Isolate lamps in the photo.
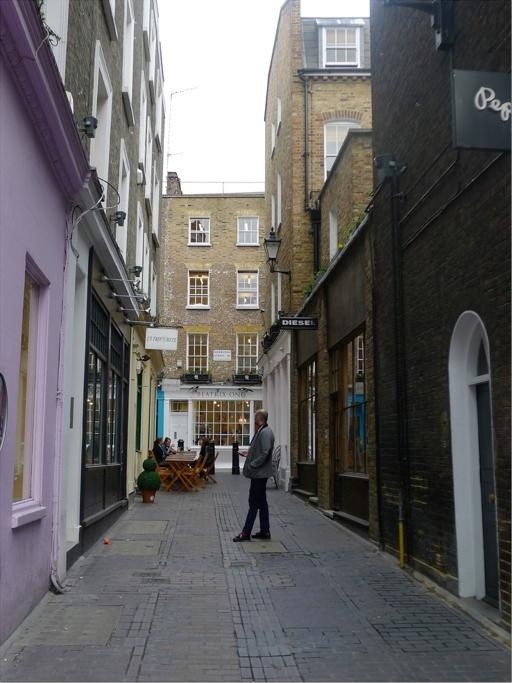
[263,226,290,280]
[76,114,99,143]
[109,210,127,226]
[138,354,152,362]
[101,265,157,328]
[373,153,403,172]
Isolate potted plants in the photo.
[231,370,260,382]
[264,320,279,346]
[182,368,212,381]
[137,458,161,503]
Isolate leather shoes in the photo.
[252,531,270,539]
[233,532,250,542]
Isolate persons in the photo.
[152,437,170,467]
[189,436,213,479]
[159,437,177,461]
[231,408,275,542]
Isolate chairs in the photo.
[148,449,219,493]
